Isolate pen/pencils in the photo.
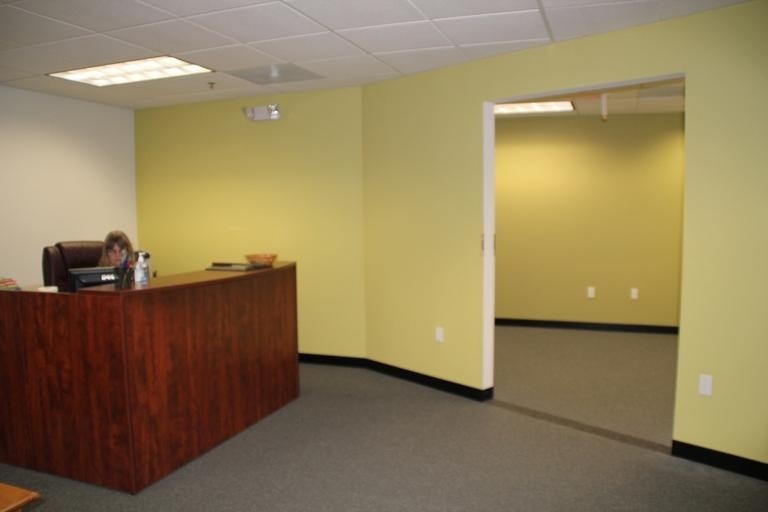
[212,262,233,266]
[120,248,134,289]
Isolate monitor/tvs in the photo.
[67,266,135,293]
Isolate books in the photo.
[206,264,273,272]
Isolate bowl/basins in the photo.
[246,253,278,269]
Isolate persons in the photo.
[98,230,134,267]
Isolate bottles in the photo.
[133,252,149,285]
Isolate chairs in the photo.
[42,241,104,291]
